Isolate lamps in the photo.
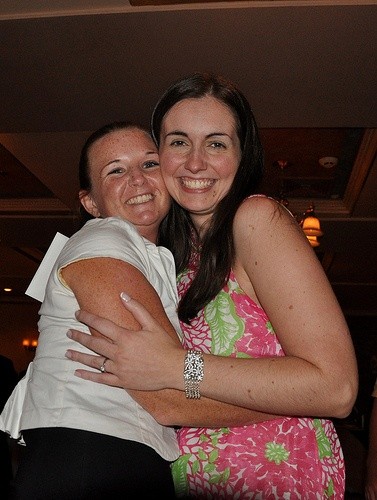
[301,200,324,247]
